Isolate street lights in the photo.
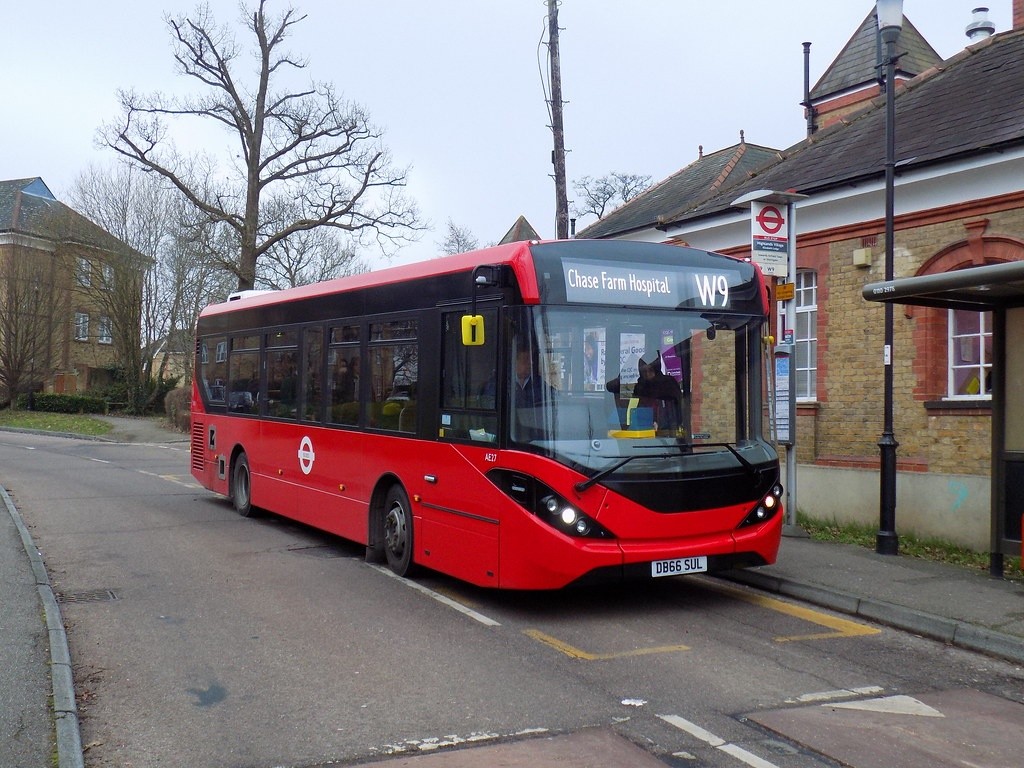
[876,0,909,555]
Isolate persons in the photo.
[586,336,599,376]
[249,342,685,447]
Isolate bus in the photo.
[189,237,785,591]
